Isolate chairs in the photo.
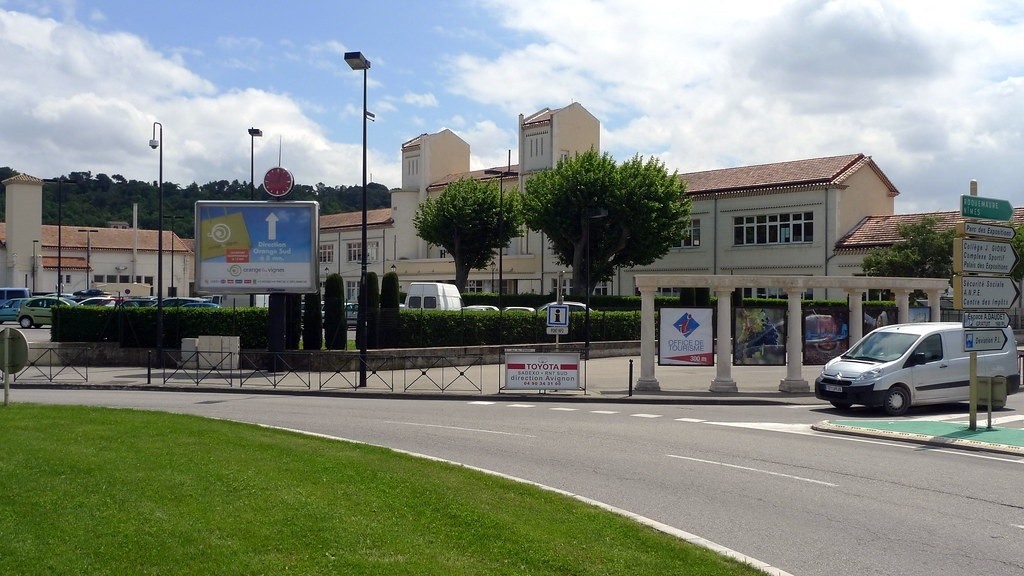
[926,339,940,359]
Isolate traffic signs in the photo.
[952,194,1021,352]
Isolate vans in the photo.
[815,321,1020,416]
[404,281,465,310]
[0,288,32,308]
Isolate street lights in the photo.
[78,230,98,290]
[42,179,78,298]
[163,215,184,298]
[345,51,371,387]
[32,240,39,292]
[584,208,609,359]
[248,127,263,306]
[484,169,519,344]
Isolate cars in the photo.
[344,303,360,329]
[0,298,29,324]
[16,296,79,328]
[151,295,223,307]
[300,301,326,329]
[538,301,594,312]
[67,295,165,307]
[504,307,535,312]
[462,305,499,311]
